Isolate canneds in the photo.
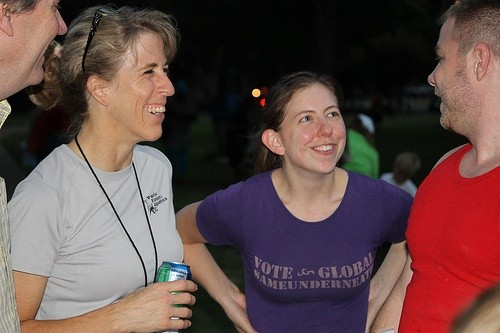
[152,260,192,321]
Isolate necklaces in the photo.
[74,132,158,288]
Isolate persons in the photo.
[176,71,419,333]
[369,0,500,333]
[0,0,69,333]
[341,109,419,199]
[4,4,198,332]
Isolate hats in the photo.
[359,114,374,134]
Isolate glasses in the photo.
[82,6,121,80]
